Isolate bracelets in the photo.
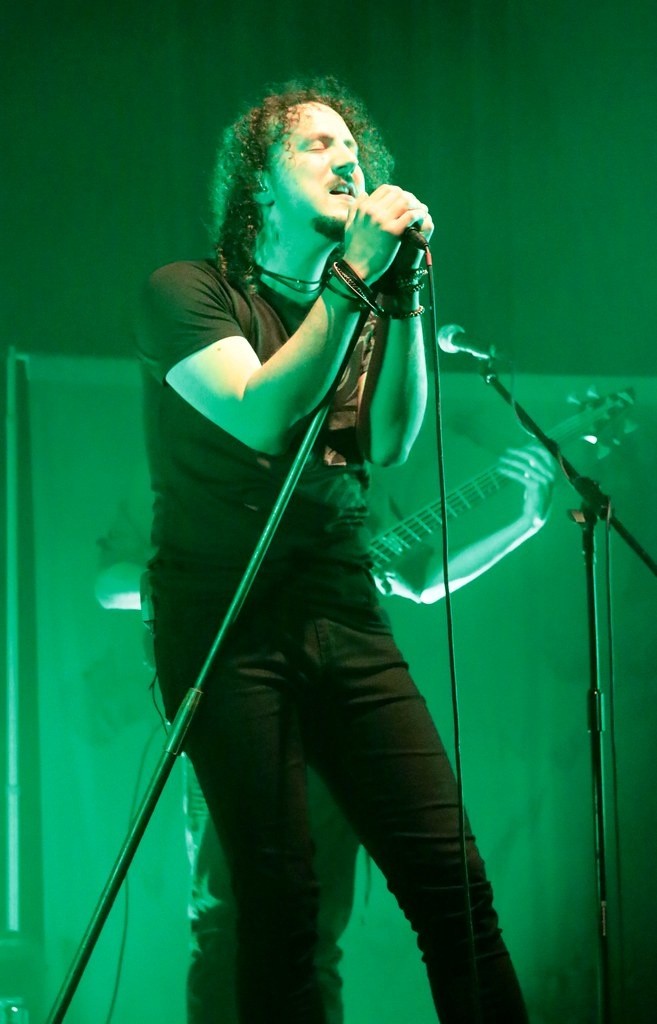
[375,268,427,320]
[326,259,375,307]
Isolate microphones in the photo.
[438,324,494,362]
[353,192,428,250]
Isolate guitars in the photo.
[140,383,642,675]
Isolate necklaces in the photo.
[259,267,324,294]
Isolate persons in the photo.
[95,443,559,1024]
[132,93,532,1024]
